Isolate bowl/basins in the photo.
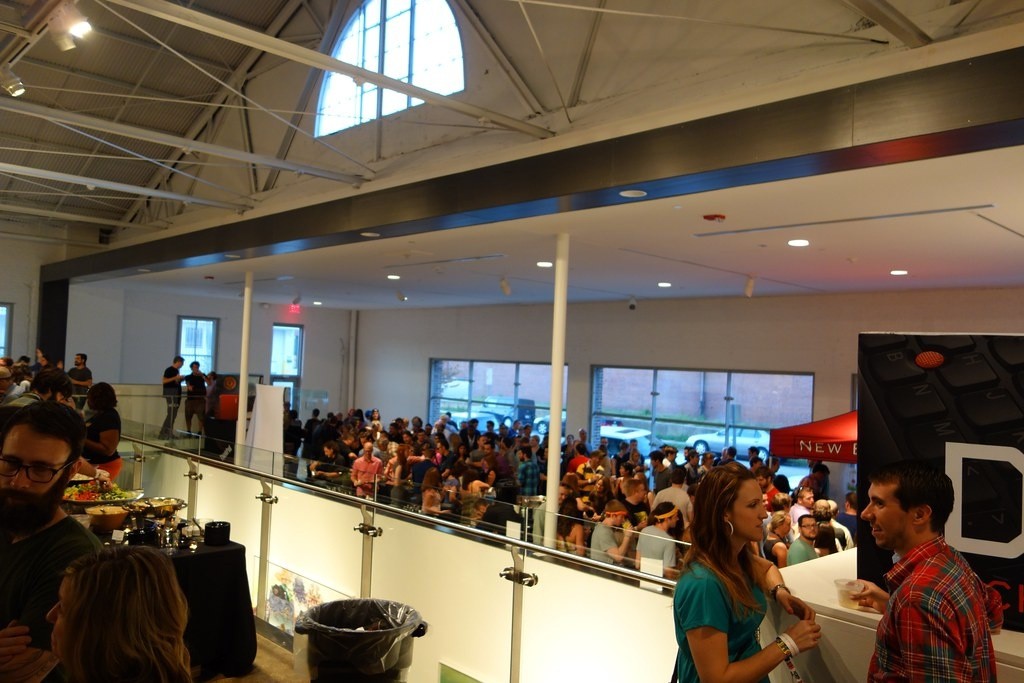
[204,521,230,545]
[60,491,144,515]
[85,505,129,529]
[122,496,187,519]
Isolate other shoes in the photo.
[184,433,191,437]
[195,432,201,438]
[158,434,178,439]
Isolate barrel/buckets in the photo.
[219,395,237,419]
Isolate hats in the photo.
[686,450,703,457]
[365,410,373,416]
[0,366,11,378]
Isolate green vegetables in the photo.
[63,482,131,499]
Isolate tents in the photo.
[769,410,858,469]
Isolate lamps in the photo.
[743,276,756,298]
[45,2,93,51]
[499,278,512,296]
[395,290,407,301]
[0,61,25,97]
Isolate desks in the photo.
[88,516,257,676]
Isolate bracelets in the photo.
[95,470,100,479]
[776,633,802,683]
[772,585,791,602]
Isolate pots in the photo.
[66,479,91,487]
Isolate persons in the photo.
[0,349,123,481]
[748,446,858,567]
[185,361,216,439]
[159,356,185,440]
[533,429,737,592]
[673,461,821,683]
[47,546,193,683]
[843,460,998,683]
[0,401,102,683]
[284,401,549,548]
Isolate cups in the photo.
[622,518,633,530]
[639,512,647,521]
[835,579,865,609]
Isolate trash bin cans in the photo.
[295,597,428,683]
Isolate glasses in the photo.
[0,458,76,483]
[800,523,818,529]
